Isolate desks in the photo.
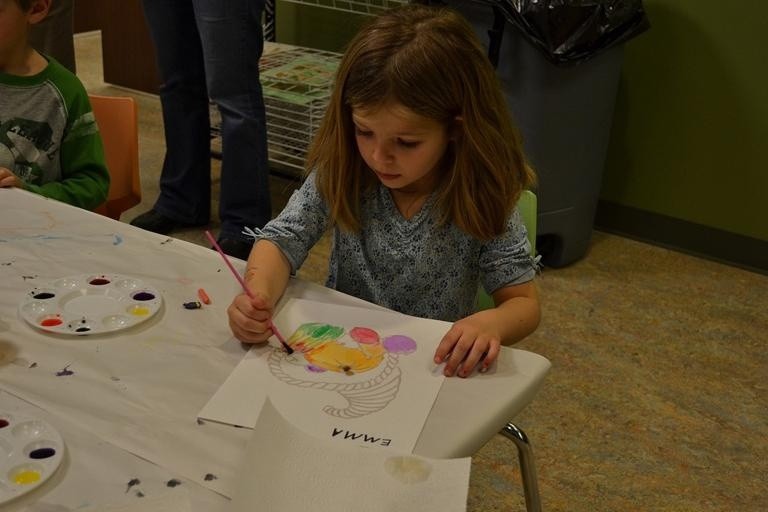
[0,186,551,512]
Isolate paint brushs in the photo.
[205,231,294,354]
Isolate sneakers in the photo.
[210,229,254,264]
[129,209,213,234]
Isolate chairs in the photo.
[87,94,142,221]
[466,191,542,512]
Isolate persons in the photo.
[128,0,274,262]
[227,9,541,377]
[0,0,113,211]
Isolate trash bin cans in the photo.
[447,0,652,269]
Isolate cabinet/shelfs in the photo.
[210,42,344,171]
